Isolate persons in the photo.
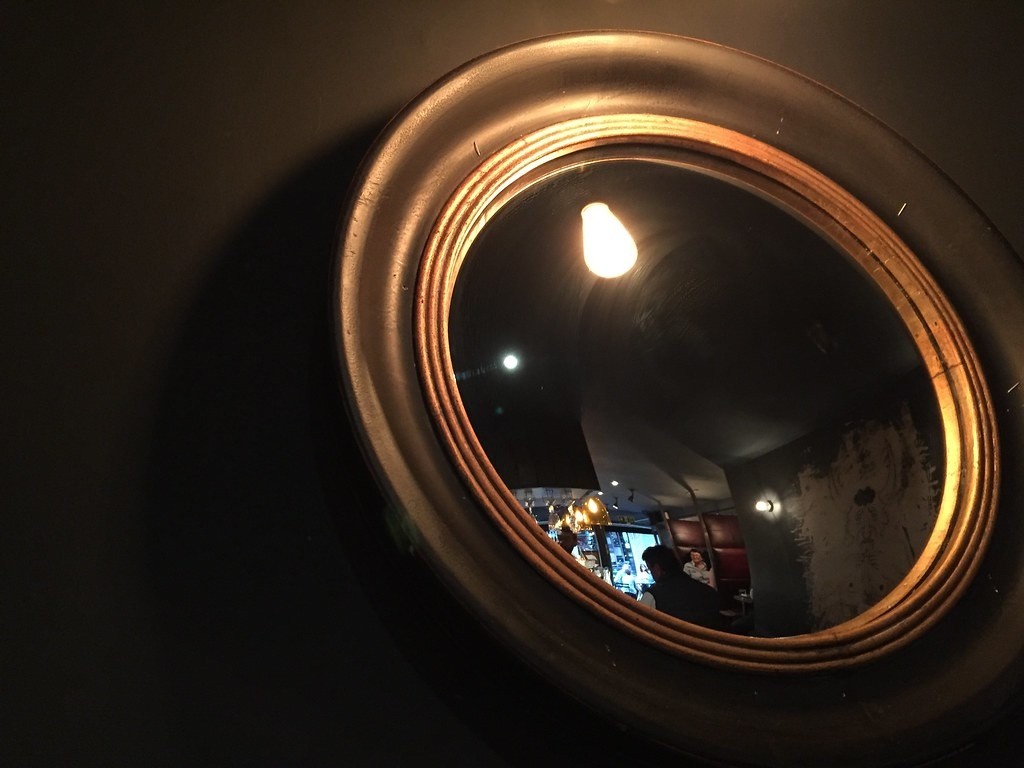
[639,544,721,631]
[613,562,655,601]
[701,549,717,590]
[683,548,708,584]
